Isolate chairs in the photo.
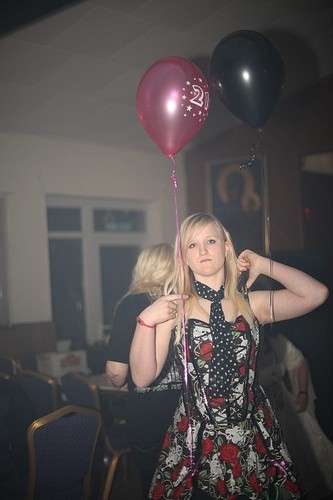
[0,358,144,500]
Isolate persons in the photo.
[105,243,182,500]
[128,212,329,500]
[256,332,333,500]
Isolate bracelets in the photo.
[299,391,307,394]
[136,316,156,328]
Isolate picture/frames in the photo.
[204,154,271,259]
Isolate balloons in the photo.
[209,29,284,129]
[136,57,211,158]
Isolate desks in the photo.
[28,349,95,402]
[85,368,182,396]
